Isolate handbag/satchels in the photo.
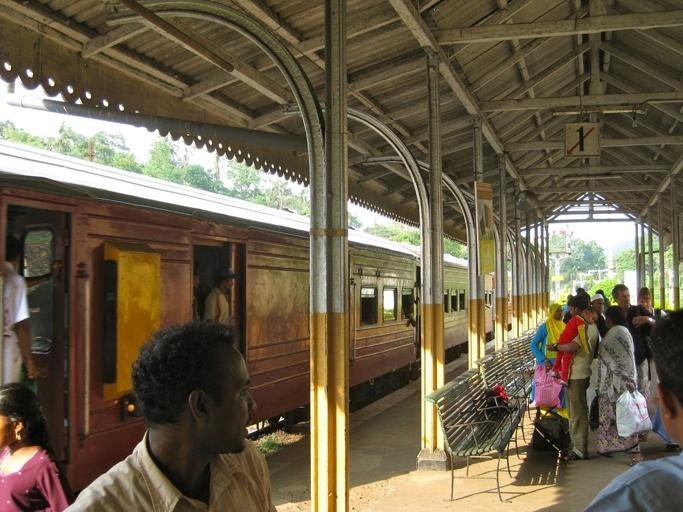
[533,416,570,449]
[534,365,563,409]
[589,397,599,431]
[616,389,652,437]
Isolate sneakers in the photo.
[563,452,581,461]
[629,454,642,465]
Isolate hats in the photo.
[217,267,239,279]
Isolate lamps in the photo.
[632,105,638,128]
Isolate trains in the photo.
[0,137,494,500]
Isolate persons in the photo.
[0,381,69,511]
[204,267,234,327]
[530,284,683,462]
[582,308,683,512]
[193,266,199,320]
[0,234,61,390]
[62,319,277,511]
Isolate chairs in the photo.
[423,326,536,502]
